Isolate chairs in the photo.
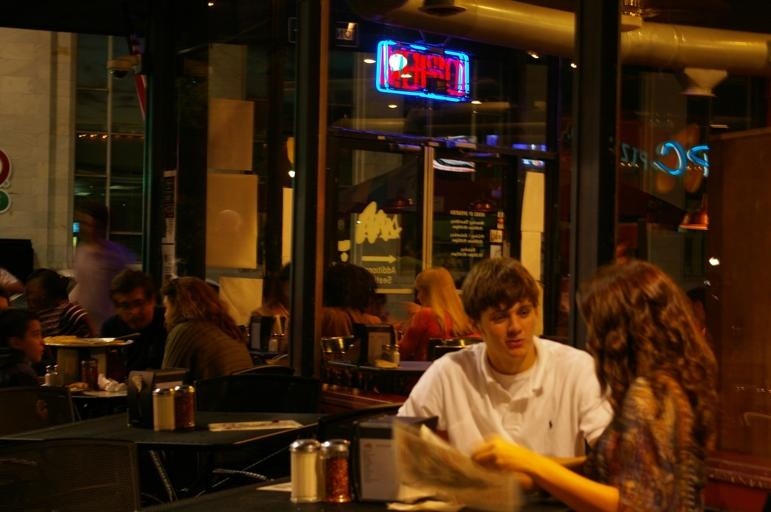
[317,401,438,443]
[351,323,399,364]
[230,363,297,376]
[190,374,324,413]
[0,384,76,437]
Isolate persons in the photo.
[468,259,721,512]
[616,244,634,259]
[2,208,398,512]
[399,266,472,359]
[394,257,606,459]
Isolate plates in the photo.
[43,337,134,347]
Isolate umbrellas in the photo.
[334,141,690,225]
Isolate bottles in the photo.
[152,387,176,432]
[268,334,278,352]
[320,439,351,504]
[53,364,64,387]
[278,334,288,353]
[391,344,400,366]
[174,385,195,428]
[44,365,55,385]
[289,439,322,504]
[81,358,98,388]
[380,345,391,362]
